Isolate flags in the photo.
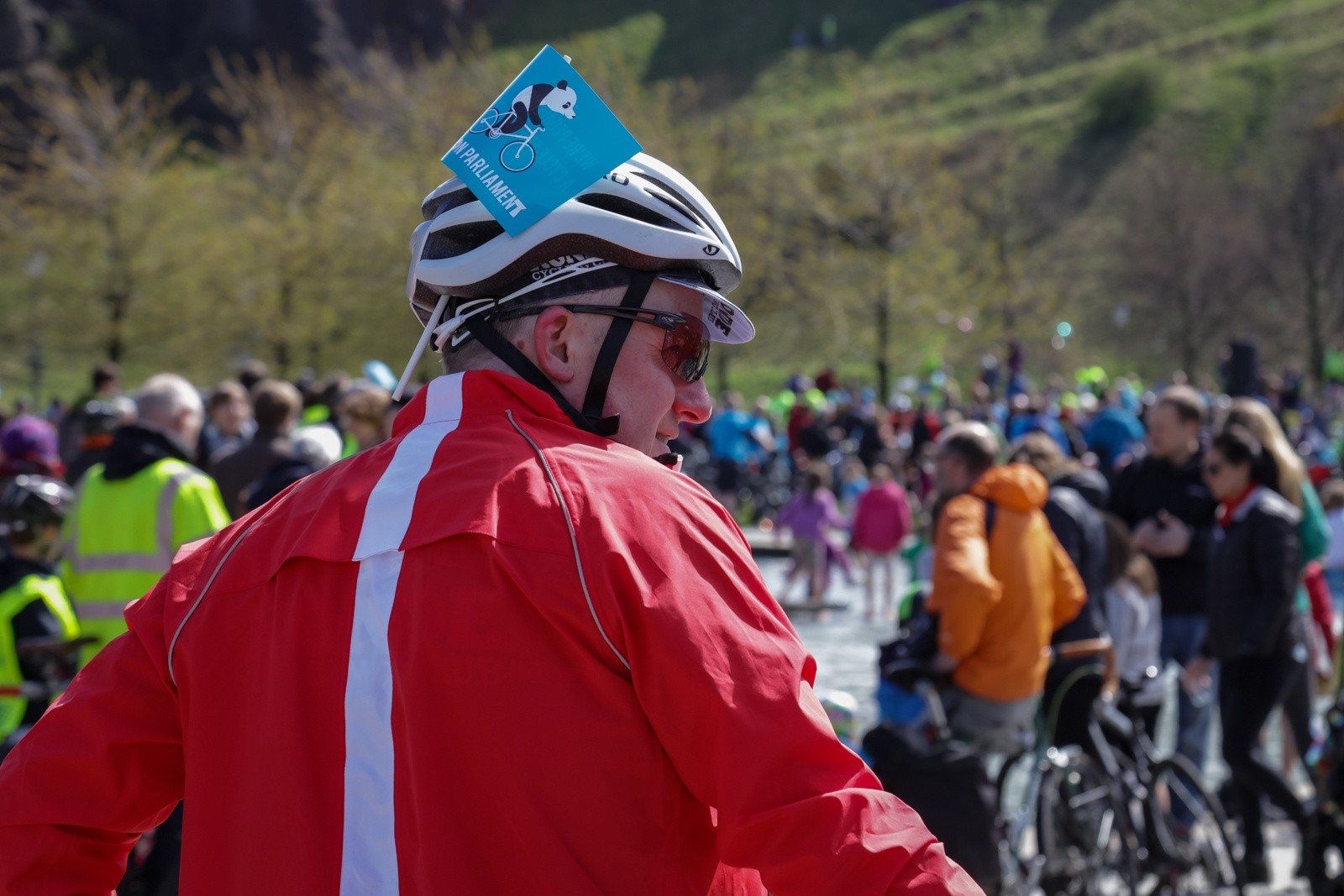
[440,44,644,237]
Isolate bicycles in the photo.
[857,625,1344,896]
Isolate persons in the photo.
[1,152,984,896]
[667,335,1344,896]
[1,360,411,896]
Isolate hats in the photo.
[436,250,755,345]
[0,414,61,472]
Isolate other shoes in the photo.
[1213,855,1269,886]
[1293,848,1312,879]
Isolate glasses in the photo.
[498,305,712,384]
[1201,458,1230,478]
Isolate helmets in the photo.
[0,473,74,545]
[407,151,742,351]
